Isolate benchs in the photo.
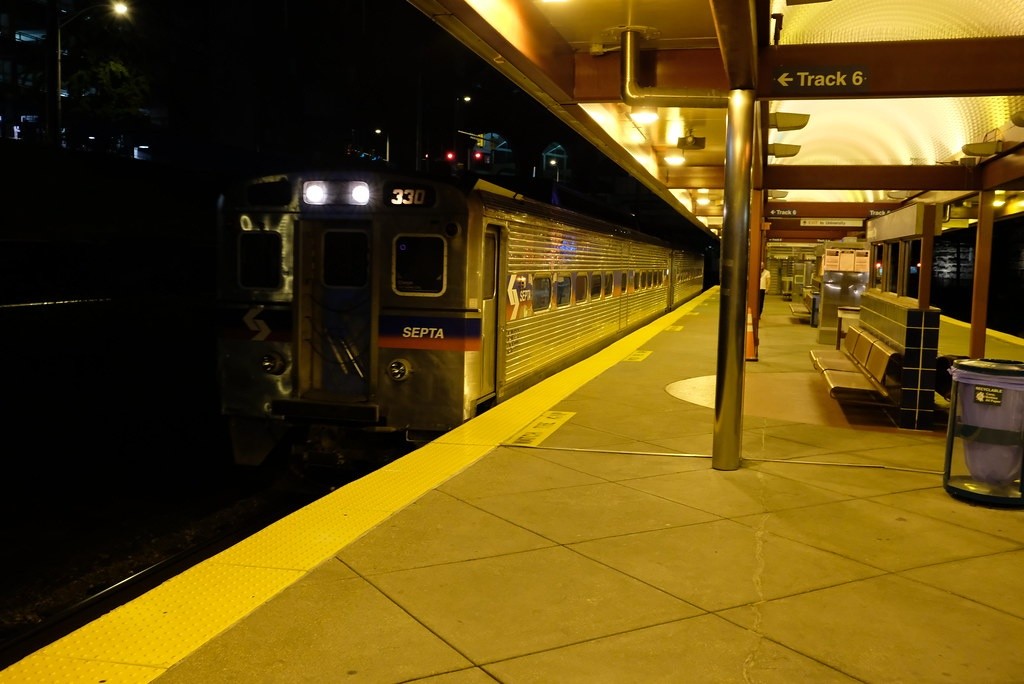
[790,293,816,325]
[810,326,902,410]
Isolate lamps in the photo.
[677,136,706,151]
[768,143,801,158]
[886,192,905,200]
[769,112,810,132]
[961,140,1003,157]
[767,190,788,198]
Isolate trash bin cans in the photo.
[941,355,1024,511]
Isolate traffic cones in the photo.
[744,306,758,362]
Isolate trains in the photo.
[215,168,703,450]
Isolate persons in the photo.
[759,262,771,319]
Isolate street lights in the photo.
[55,1,130,120]
[375,127,390,162]
